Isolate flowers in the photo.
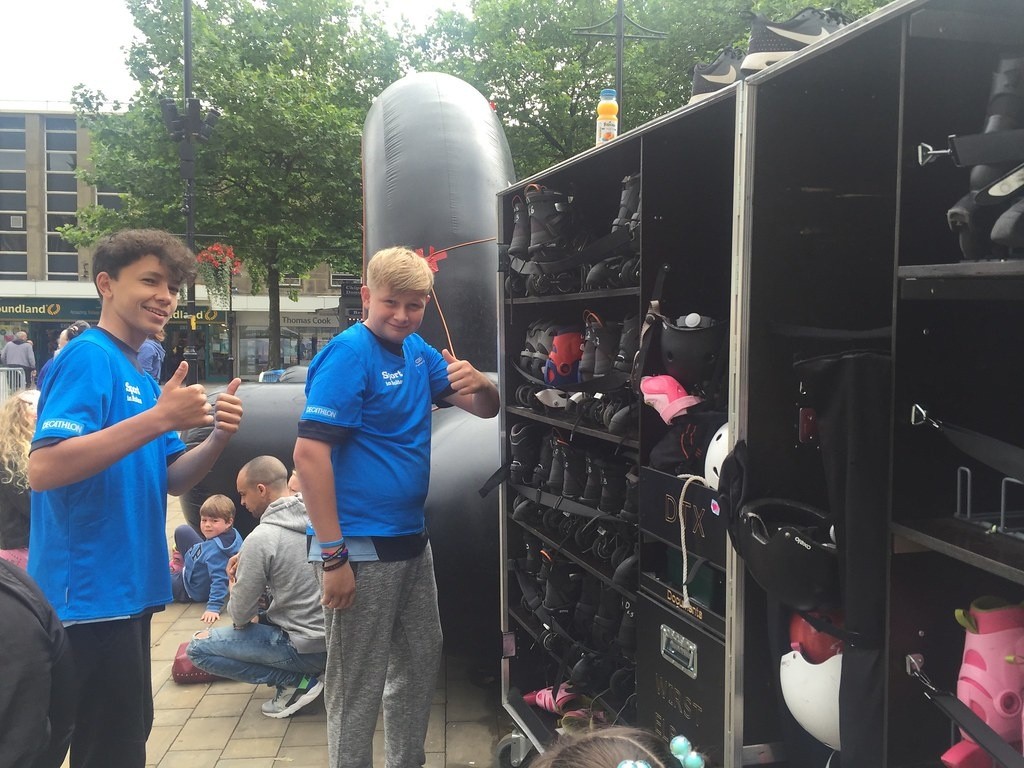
[198,242,242,311]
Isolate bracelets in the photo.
[318,537,348,571]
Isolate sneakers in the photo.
[507,5,1024,768]
[261,674,324,719]
[173,544,185,565]
[169,560,183,574]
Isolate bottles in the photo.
[596,88,619,148]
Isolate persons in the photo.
[25,228,243,768]
[287,470,301,496]
[292,245,501,768]
[137,321,165,384]
[0,558,77,768]
[0,390,43,571]
[166,495,243,623]
[56,321,90,350]
[1,331,37,393]
[186,456,327,719]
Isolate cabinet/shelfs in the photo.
[492,0,1024,768]
